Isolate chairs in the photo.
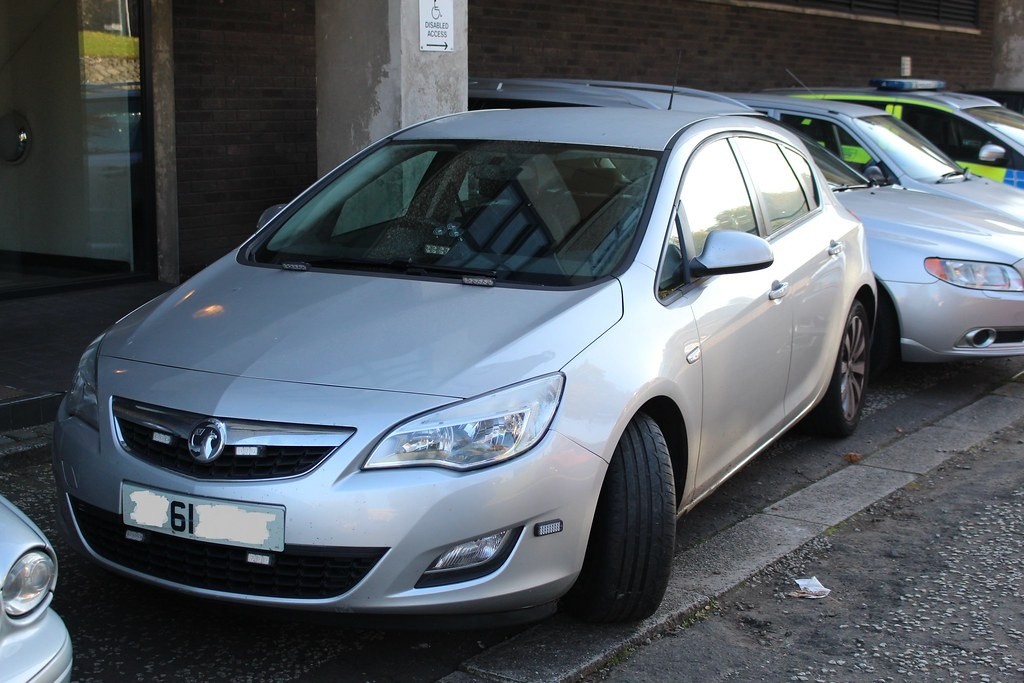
[458,155,566,257]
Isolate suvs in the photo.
[468,74,1023,383]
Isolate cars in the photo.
[46,103,880,633]
[710,91,1022,224]
[763,89,1023,190]
[0,495,75,683]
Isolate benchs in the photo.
[537,163,643,250]
